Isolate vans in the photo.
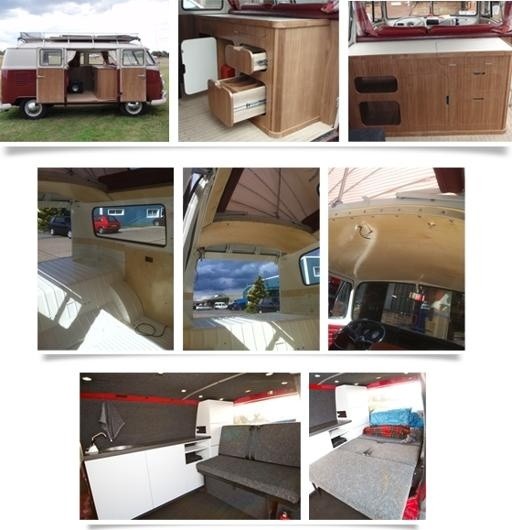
[214,302,229,310]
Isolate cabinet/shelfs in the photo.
[179,12,337,139]
[92,65,118,99]
[83,435,212,519]
[347,36,512,138]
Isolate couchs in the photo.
[198,421,300,519]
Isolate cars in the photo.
[47,215,72,239]
[255,302,280,313]
[93,215,121,234]
[195,304,213,310]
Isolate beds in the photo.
[309,433,423,520]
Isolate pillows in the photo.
[364,408,424,442]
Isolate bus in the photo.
[1,32,166,119]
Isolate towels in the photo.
[100,400,124,440]
